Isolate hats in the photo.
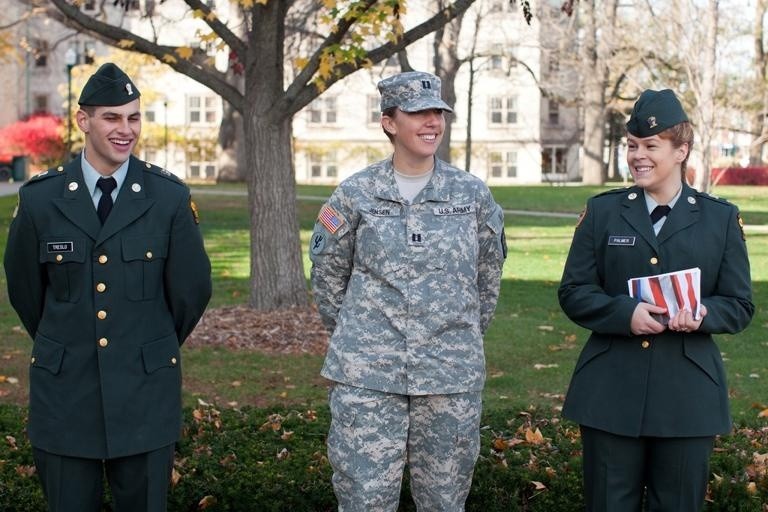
[378,71,454,113]
[78,63,140,107]
[627,89,689,138]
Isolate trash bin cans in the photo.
[12,156,25,181]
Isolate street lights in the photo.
[62,46,80,165]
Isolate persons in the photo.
[309,70,509,511]
[3,62,213,512]
[556,87,756,512]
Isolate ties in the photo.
[96,177,117,224]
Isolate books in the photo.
[627,267,702,328]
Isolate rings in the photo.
[680,327,688,332]
